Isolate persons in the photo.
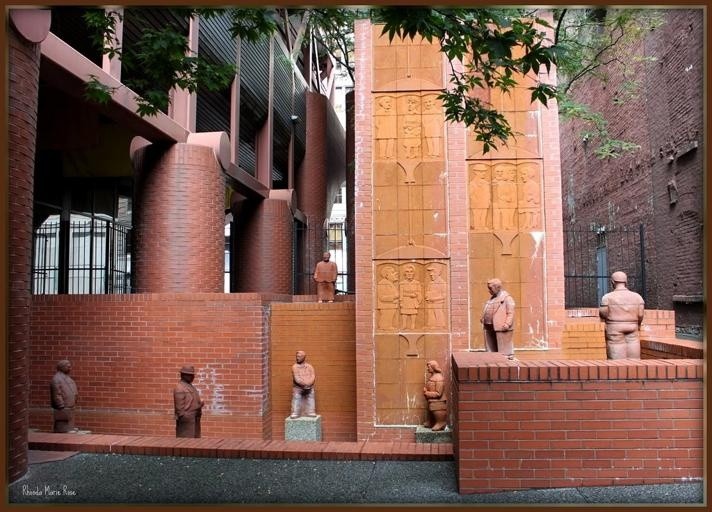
[175,363,204,437]
[315,252,338,304]
[479,277,515,353]
[599,271,644,360]
[50,358,80,432]
[423,360,449,431]
[290,348,317,417]
